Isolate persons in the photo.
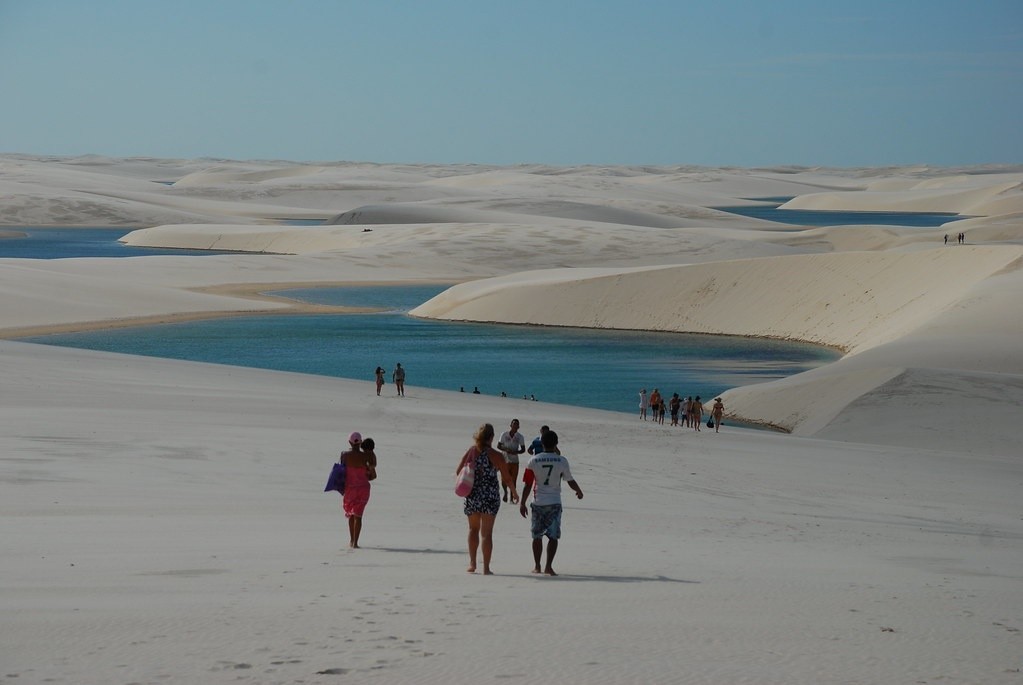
[374,366,385,395]
[527,425,560,455]
[649,389,660,422]
[497,419,525,503]
[944,235,947,244]
[659,393,704,431]
[473,387,480,394]
[340,432,377,548]
[456,424,519,576]
[501,392,506,397]
[711,397,724,433]
[460,387,465,392]
[958,233,964,244]
[530,395,534,401]
[393,363,405,395]
[639,389,648,421]
[523,394,526,399]
[520,431,583,576]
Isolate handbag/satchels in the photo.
[323,451,346,496]
[706,419,714,428]
[454,446,475,497]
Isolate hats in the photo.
[695,396,702,400]
[714,398,722,402]
[349,432,363,444]
